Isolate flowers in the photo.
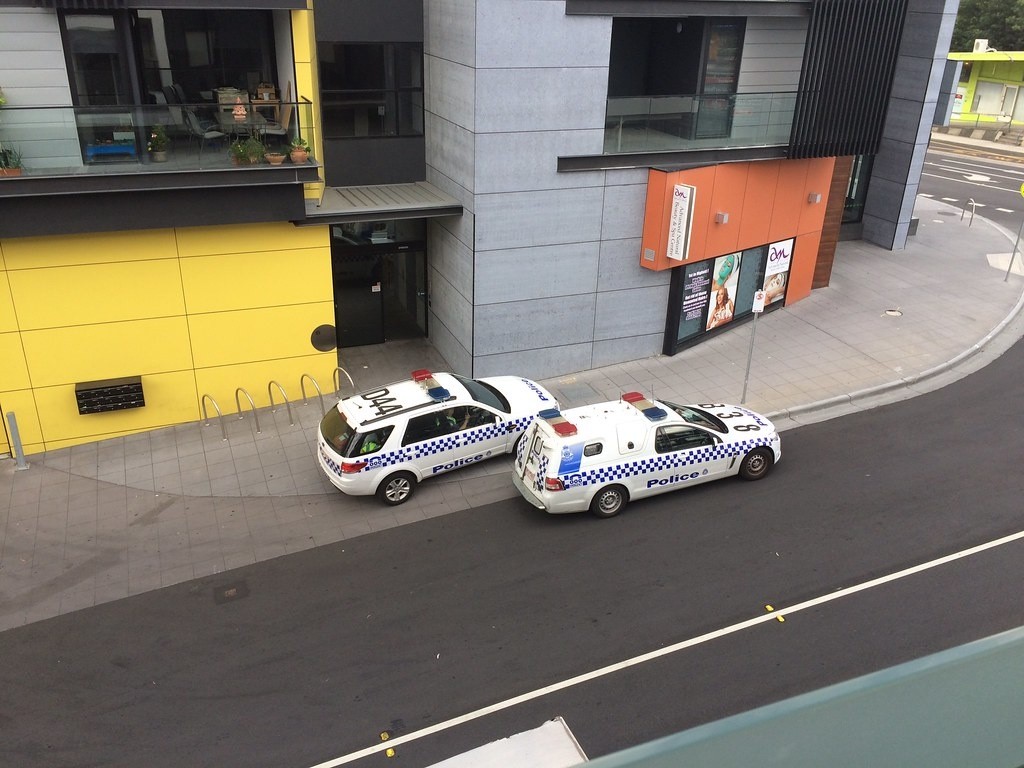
[227,136,266,165]
[147,124,173,152]
[233,154,241,165]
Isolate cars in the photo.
[316,367,560,507]
[515,392,782,519]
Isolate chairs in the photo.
[256,82,293,146]
[162,82,231,161]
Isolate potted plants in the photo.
[286,137,311,164]
[0,139,30,177]
[264,144,286,165]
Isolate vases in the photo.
[153,149,167,163]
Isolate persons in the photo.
[763,273,786,306]
[706,253,739,330]
[434,408,470,431]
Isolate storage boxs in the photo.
[257,83,276,99]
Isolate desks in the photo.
[212,88,250,113]
[215,111,267,147]
[252,98,282,122]
